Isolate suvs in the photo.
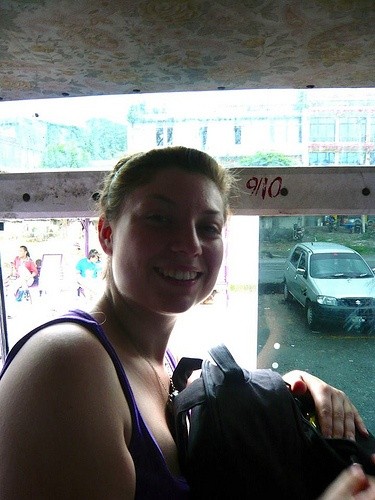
[282,242,375,334]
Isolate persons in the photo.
[6,246,38,302]
[72,248,101,311]
[0,146,375,500]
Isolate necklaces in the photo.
[105,295,180,398]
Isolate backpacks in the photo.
[163,342,375,500]
